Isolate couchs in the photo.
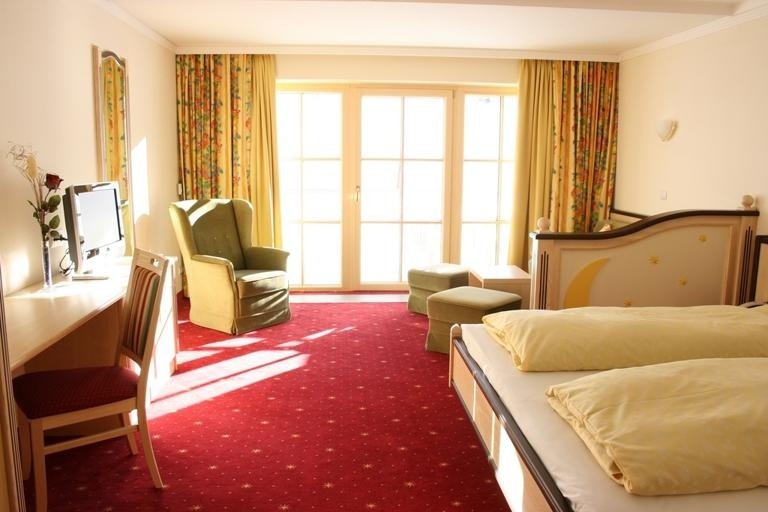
[528,195,758,310]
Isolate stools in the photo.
[424,285,522,355]
[407,262,467,315]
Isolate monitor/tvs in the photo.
[63,181,124,280]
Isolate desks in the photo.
[3,253,181,437]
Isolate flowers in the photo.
[6,140,64,281]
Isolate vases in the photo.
[41,240,53,288]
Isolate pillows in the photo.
[598,223,612,233]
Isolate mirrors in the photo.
[92,44,131,208]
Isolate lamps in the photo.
[656,117,677,143]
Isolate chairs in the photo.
[12,246,169,512]
[169,198,292,336]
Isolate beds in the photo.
[447,234,768,512]
